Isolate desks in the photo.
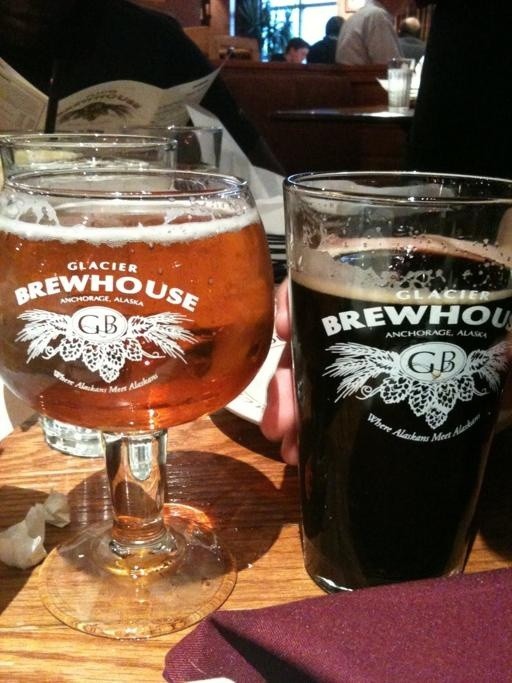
[270,104,415,143]
[1,413,511,683]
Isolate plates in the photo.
[224,317,288,425]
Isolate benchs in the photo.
[218,64,415,173]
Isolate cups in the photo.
[384,56,415,113]
[284,167,512,598]
[3,126,221,462]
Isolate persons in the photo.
[258,0,511,470]
[0,0,292,180]
[305,14,344,61]
[269,37,310,66]
[334,0,403,63]
[394,17,427,60]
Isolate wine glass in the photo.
[3,174,270,642]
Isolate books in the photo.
[0,51,233,140]
